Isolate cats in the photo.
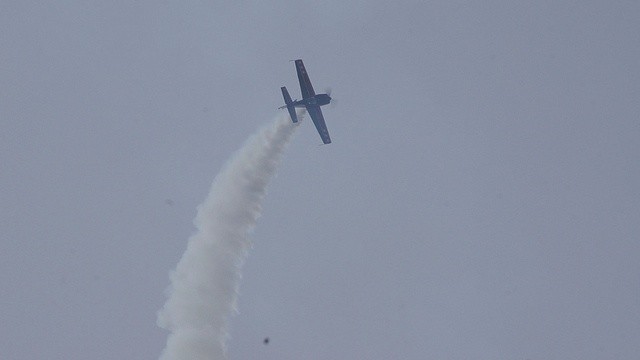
[278,58,335,145]
